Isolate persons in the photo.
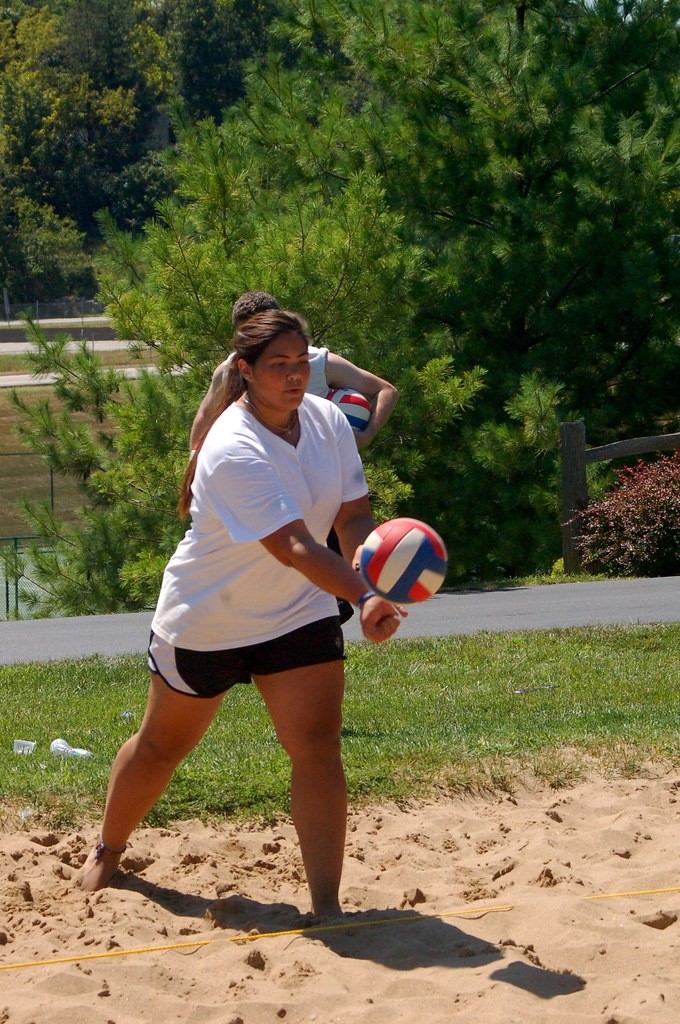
[189,290,398,624]
[80,308,408,924]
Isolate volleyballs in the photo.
[361,519,447,603]
[325,388,371,433]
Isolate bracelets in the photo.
[358,590,377,609]
